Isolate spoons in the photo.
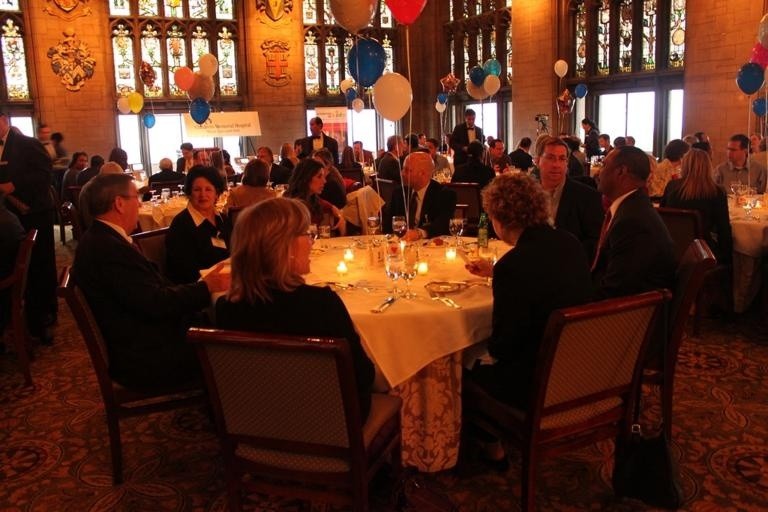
[373,296,394,314]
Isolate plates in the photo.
[425,282,459,293]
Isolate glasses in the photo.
[294,228,317,243]
[112,192,142,204]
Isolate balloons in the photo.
[370,73,414,122]
[117,51,218,127]
[554,59,588,117]
[737,15,768,115]
[383,0,427,25]
[331,0,379,32]
[338,76,367,111]
[350,39,386,87]
[436,57,503,113]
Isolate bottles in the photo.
[366,218,383,246]
[478,212,487,248]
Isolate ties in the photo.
[590,210,611,272]
[409,191,418,229]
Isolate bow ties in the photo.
[310,136,321,139]
[466,127,473,130]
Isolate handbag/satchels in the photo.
[612,434,681,512]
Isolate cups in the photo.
[590,155,605,166]
[146,184,186,209]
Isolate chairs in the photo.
[653,207,702,254]
[626,238,716,447]
[454,204,470,236]
[57,265,209,484]
[188,327,403,512]
[360,166,480,220]
[0,227,37,385]
[48,180,288,247]
[129,225,169,286]
[463,288,672,512]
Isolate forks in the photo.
[430,291,462,309]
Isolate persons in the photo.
[0,112,767,500]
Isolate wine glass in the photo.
[449,220,463,249]
[319,225,330,253]
[403,246,419,299]
[727,184,761,224]
[392,217,407,245]
[306,225,318,257]
[386,254,404,302]
